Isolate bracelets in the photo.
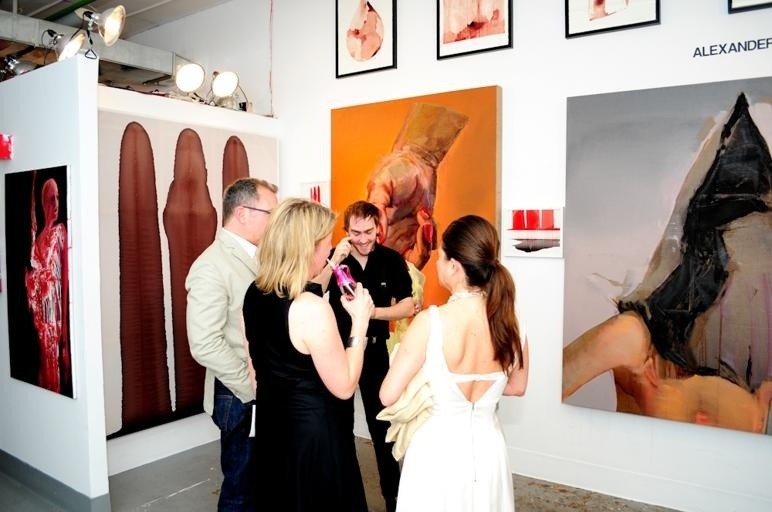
[344,334,368,351]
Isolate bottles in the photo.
[328,262,360,303]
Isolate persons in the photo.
[379,214,529,512]
[241,197,375,512]
[562,91,772,435]
[24,177,68,399]
[366,105,471,271]
[308,202,414,512]
[185,178,277,475]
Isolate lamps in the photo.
[37,29,75,66]
[208,71,250,109]
[0,54,39,75]
[79,5,126,58]
[171,64,206,101]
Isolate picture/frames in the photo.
[437,0,512,59]
[564,0,660,38]
[335,1,397,78]
[727,0,772,13]
